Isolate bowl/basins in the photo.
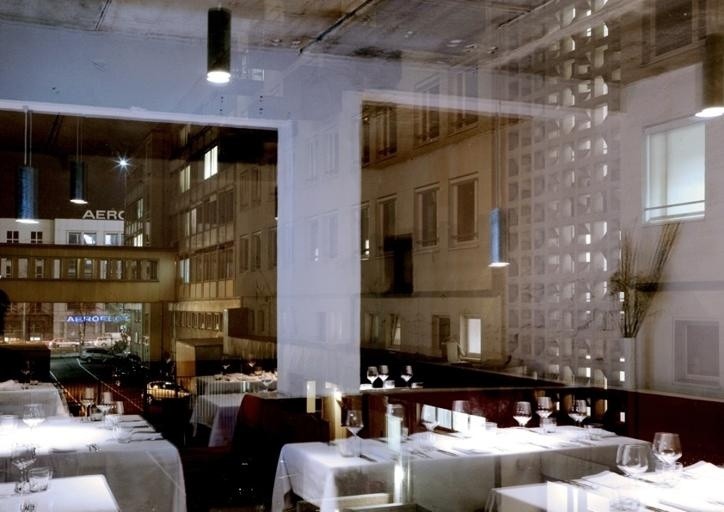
[146,380,176,391]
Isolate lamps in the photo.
[16,105,40,226]
[488,49,513,272]
[692,5,723,119]
[204,1,233,86]
[69,116,91,208]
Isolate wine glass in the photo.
[613,428,685,511]
[214,357,274,397]
[13,369,43,390]
[346,364,595,457]
[0,389,123,512]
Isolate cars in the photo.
[49,331,192,404]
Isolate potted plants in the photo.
[606,220,683,391]
